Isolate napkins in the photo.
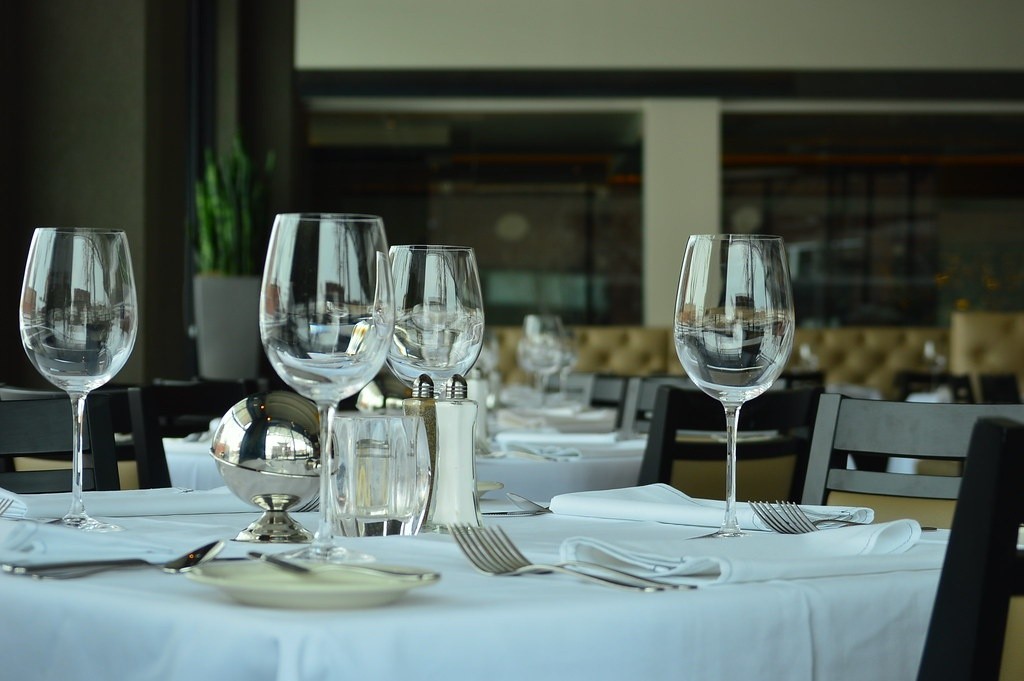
[534,444,583,464]
[0,522,178,568]
[558,537,731,592]
[549,482,875,532]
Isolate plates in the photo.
[188,564,441,611]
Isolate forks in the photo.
[477,526,699,589]
[747,500,800,534]
[1,498,14,516]
[446,523,665,591]
[775,499,856,533]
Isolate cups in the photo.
[329,412,420,537]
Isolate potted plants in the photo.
[193,124,278,378]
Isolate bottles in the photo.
[426,373,486,533]
[402,374,438,526]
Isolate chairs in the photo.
[146,377,267,486]
[0,382,145,494]
[896,366,975,403]
[621,376,694,432]
[915,416,1024,681]
[800,392,1023,599]
[585,372,643,429]
[977,372,1020,404]
[638,383,826,503]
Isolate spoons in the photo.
[506,492,549,514]
[1,541,224,576]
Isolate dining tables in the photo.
[160,388,647,502]
[0,492,943,681]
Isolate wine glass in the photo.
[674,232,796,542]
[384,243,486,535]
[473,315,574,414]
[20,226,137,535]
[258,212,395,567]
